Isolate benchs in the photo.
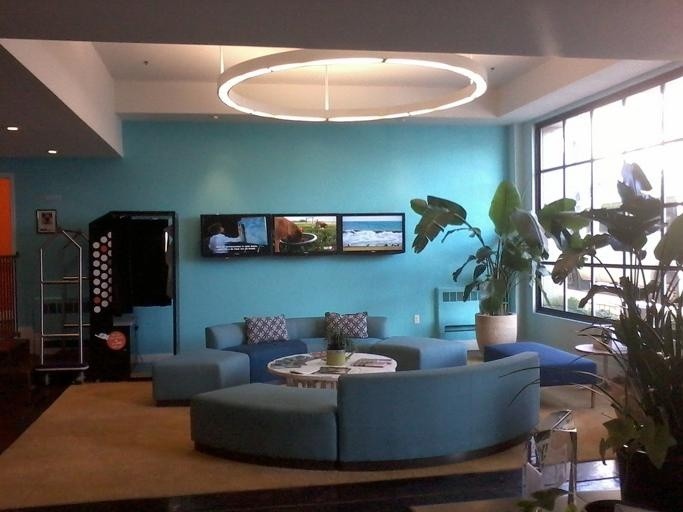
[485,340,596,408]
[0,354,42,407]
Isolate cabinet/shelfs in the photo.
[34,229,88,386]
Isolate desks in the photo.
[574,343,629,377]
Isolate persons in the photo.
[208,222,243,255]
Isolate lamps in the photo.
[217,46,487,122]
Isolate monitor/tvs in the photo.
[270,214,339,256]
[340,214,405,255]
[200,214,270,257]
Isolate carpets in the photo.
[0,350,617,512]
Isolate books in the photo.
[290,366,320,375]
[352,359,391,367]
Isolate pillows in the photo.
[324,311,369,339]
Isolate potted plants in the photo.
[525,163,683,512]
[411,179,549,353]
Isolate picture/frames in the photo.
[36,209,56,233]
